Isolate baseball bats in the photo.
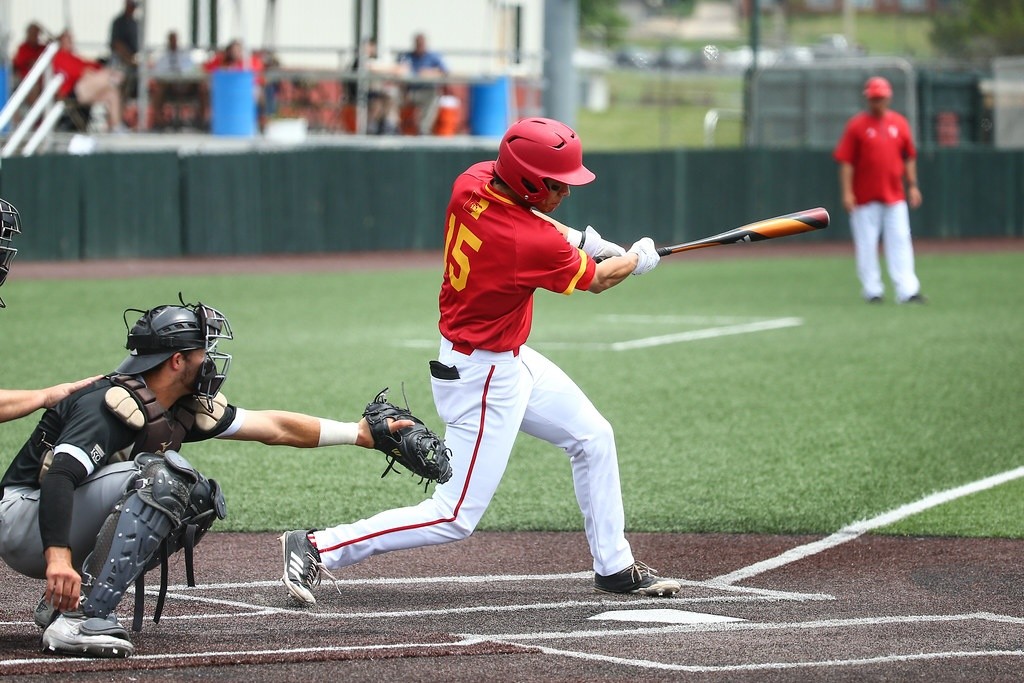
[594,205,831,264]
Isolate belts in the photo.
[452,342,519,358]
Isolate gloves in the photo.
[627,237,661,276]
[581,225,626,260]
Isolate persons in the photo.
[200,43,267,105]
[148,32,210,133]
[53,35,133,133]
[0,302,454,658]
[13,24,48,104]
[349,35,446,134]
[0,198,108,424]
[276,119,677,605]
[110,0,138,80]
[836,75,924,303]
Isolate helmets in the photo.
[0,199,17,285]
[494,118,597,206]
[116,305,213,374]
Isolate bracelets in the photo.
[579,231,586,249]
[318,419,358,447]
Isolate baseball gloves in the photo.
[360,379,455,495]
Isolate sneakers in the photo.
[34,591,88,628]
[594,560,681,595]
[42,614,135,657]
[276,528,341,605]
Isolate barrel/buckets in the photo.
[469,75,508,138]
[209,69,257,137]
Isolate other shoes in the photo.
[911,296,926,303]
[871,297,881,302]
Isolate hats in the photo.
[864,77,892,98]
